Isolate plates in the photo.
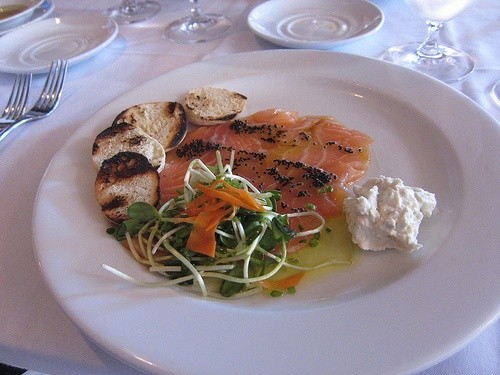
[247,1,385,49]
[31,49,500,375]
[0,0,55,36]
[0,15,119,74]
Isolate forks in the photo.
[0,72,32,135]
[0,59,68,142]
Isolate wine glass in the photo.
[378,1,475,82]
[104,0,161,24]
[163,0,233,43]
[461,68,500,123]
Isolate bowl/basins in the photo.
[0,0,45,30]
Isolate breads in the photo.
[91,122,166,176]
[94,152,160,225]
[112,101,188,152]
[179,87,248,125]
